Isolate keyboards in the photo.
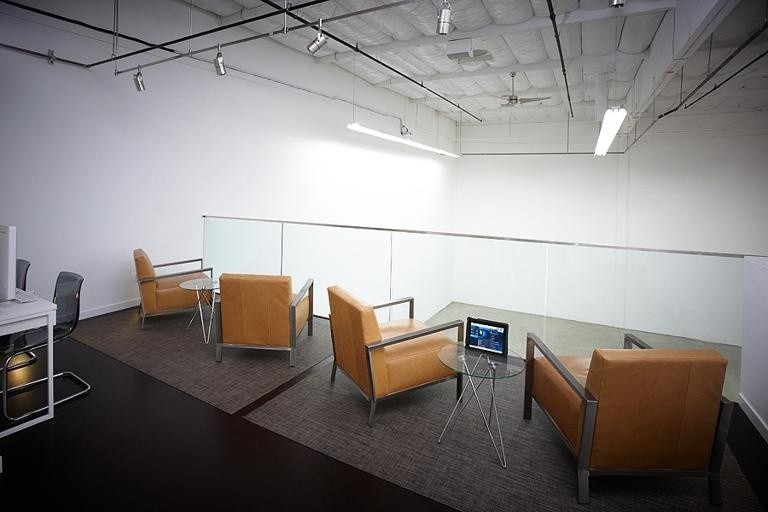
[14,286,38,303]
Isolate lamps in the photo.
[132,64,146,92]
[593,20,627,157]
[608,1,624,9]
[345,52,465,159]
[214,42,227,76]
[307,18,327,54]
[436,3,451,36]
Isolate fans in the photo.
[488,72,552,107]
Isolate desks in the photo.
[432,340,528,469]
[0,284,58,440]
[174,275,223,347]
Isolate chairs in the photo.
[1,255,42,379]
[323,281,465,428]
[520,322,741,510]
[209,270,319,369]
[1,271,96,424]
[130,246,216,332]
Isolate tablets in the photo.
[465,317,508,357]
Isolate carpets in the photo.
[242,348,764,511]
[63,296,335,417]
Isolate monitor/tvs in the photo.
[0,224,16,303]
